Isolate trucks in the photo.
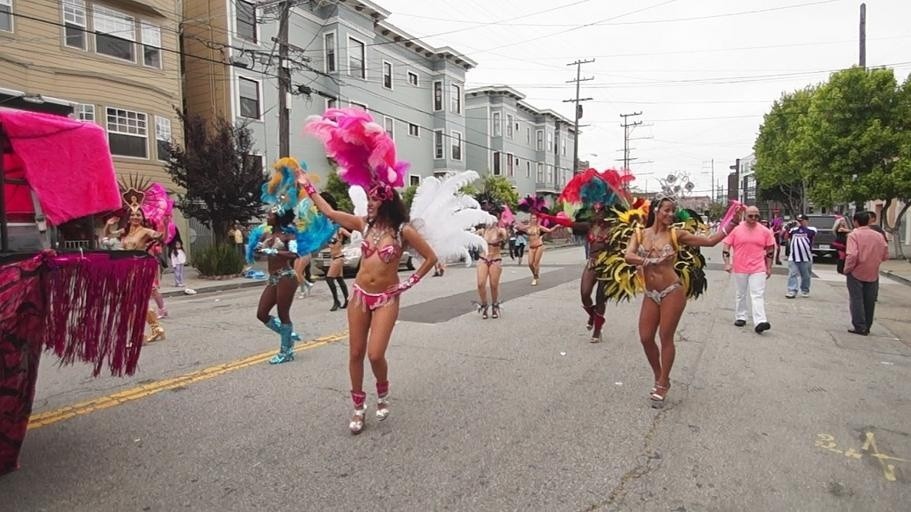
[1,87,159,476]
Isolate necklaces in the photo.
[370,225,390,246]
[129,225,143,238]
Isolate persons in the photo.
[325,226,351,313]
[866,211,890,244]
[432,262,445,278]
[293,253,315,300]
[150,278,168,321]
[512,212,565,287]
[769,217,783,267]
[469,213,511,321]
[720,205,778,336]
[228,224,245,260]
[624,193,746,405]
[168,237,189,288]
[515,230,528,266]
[780,214,818,298]
[101,205,170,348]
[252,207,302,366]
[533,201,617,345]
[842,210,891,337]
[508,229,519,261]
[831,216,852,273]
[294,168,441,438]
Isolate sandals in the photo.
[648,377,672,410]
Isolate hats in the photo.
[794,214,809,221]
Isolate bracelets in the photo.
[641,257,658,267]
[723,251,730,258]
[722,218,740,236]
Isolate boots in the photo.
[347,389,368,436]
[582,303,607,345]
[263,315,303,365]
[324,275,350,312]
[374,380,391,423]
[123,304,171,350]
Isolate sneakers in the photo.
[847,328,871,337]
[530,271,541,286]
[784,290,810,299]
[481,302,498,319]
[296,281,316,300]
[754,322,771,334]
[734,320,747,328]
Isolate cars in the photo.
[319,235,417,276]
[759,213,854,262]
[695,212,720,233]
